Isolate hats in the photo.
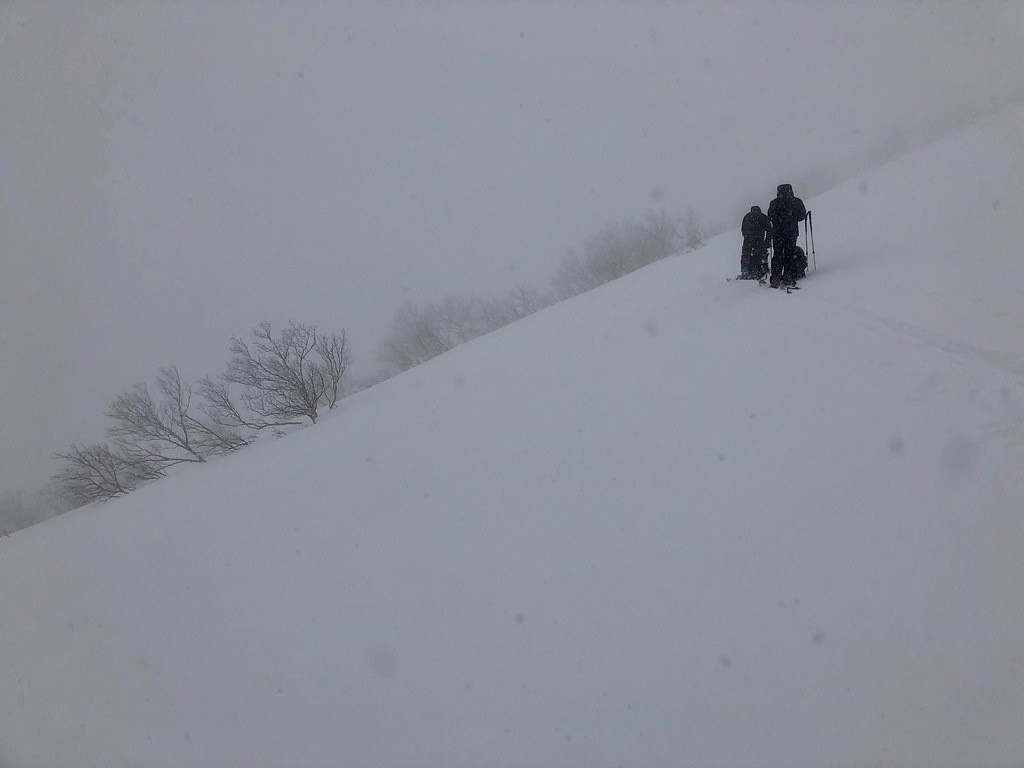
[750,205,761,213]
[776,184,793,193]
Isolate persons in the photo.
[740,184,809,290]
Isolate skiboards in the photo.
[726,274,801,294]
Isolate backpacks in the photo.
[793,246,807,278]
[750,248,769,277]
